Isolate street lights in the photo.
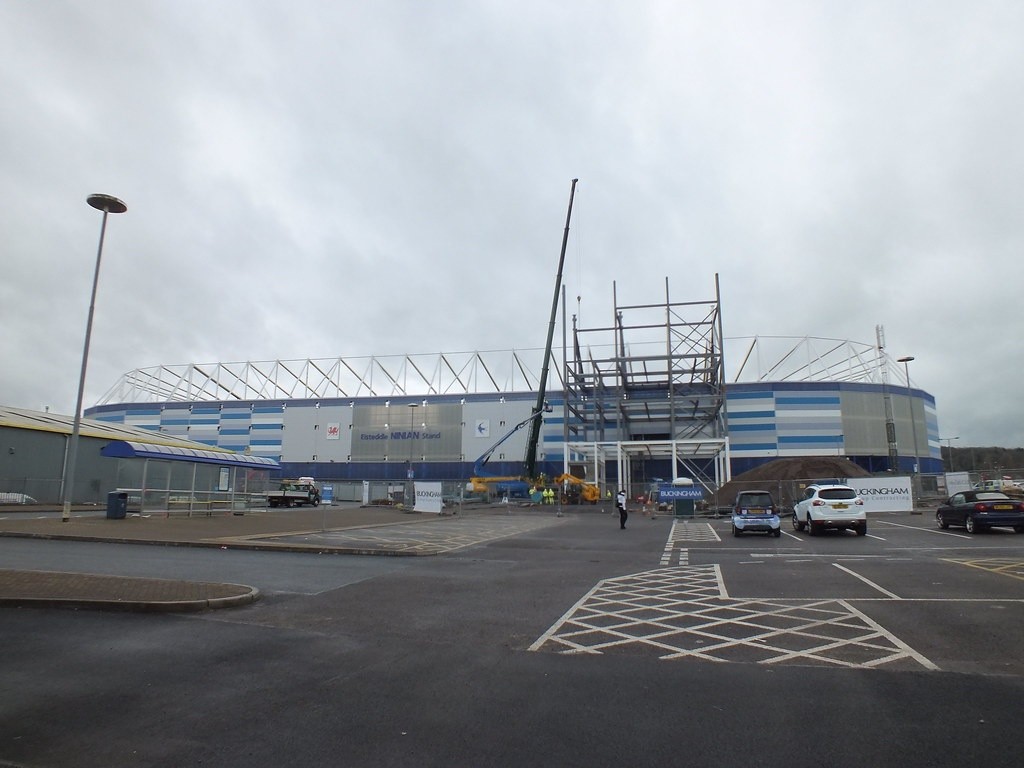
[407,402,418,513]
[936,436,960,473]
[896,357,930,507]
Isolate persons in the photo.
[543,488,549,504]
[606,489,611,497]
[616,489,628,529]
[548,488,555,505]
[529,485,535,494]
[502,491,509,503]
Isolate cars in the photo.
[966,479,1024,492]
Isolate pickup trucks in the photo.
[731,490,781,538]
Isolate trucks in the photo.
[263,481,321,507]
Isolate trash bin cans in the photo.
[107,490,128,519]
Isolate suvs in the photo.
[792,483,867,536]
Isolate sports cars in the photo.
[936,490,1024,532]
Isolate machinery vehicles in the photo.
[553,473,601,506]
[465,402,556,502]
